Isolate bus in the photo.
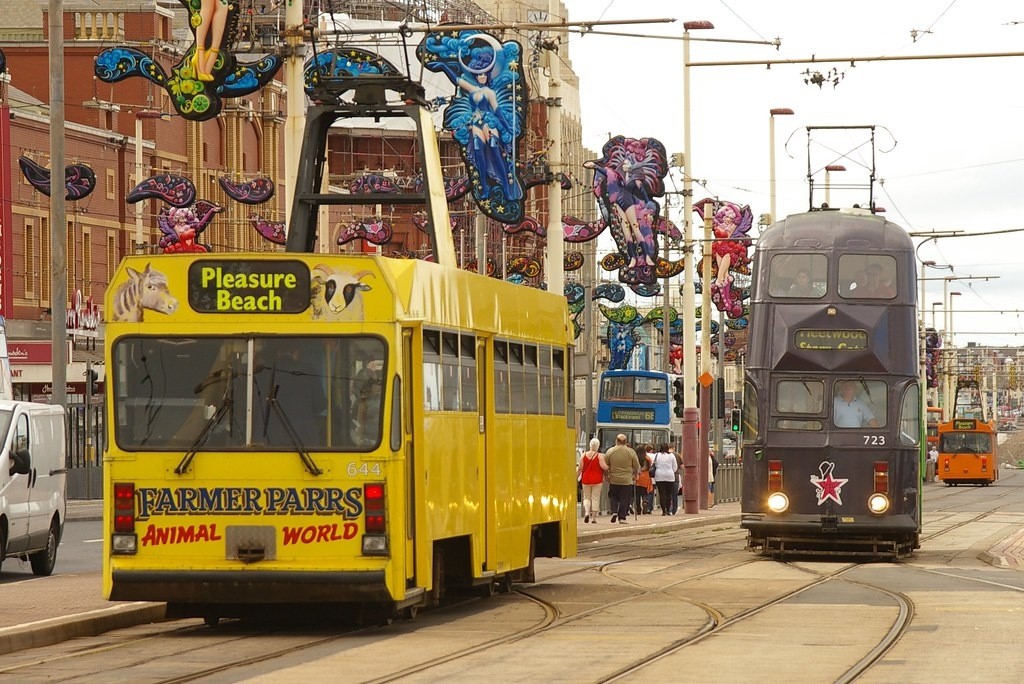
[737,123,931,563]
[925,399,944,472]
[936,378,1000,486]
[100,251,581,623]
[596,369,683,456]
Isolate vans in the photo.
[0,400,68,575]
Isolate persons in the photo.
[834,381,878,428]
[604,434,639,524]
[346,367,399,448]
[252,338,326,447]
[847,263,895,297]
[708,452,719,507]
[926,445,938,484]
[788,268,818,296]
[599,442,684,516]
[577,438,610,523]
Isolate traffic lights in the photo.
[731,409,742,433]
[674,377,685,418]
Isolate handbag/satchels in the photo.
[577,476,583,489]
[649,452,658,477]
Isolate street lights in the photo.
[930,299,943,331]
[768,105,797,227]
[822,164,848,208]
[948,291,964,420]
[682,18,717,514]
[919,260,939,478]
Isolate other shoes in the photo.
[619,519,629,524]
[584,514,589,523]
[637,507,641,515]
[643,503,647,514]
[667,512,670,516]
[662,512,665,516]
[592,520,596,523]
[648,510,652,514]
[610,513,617,523]
